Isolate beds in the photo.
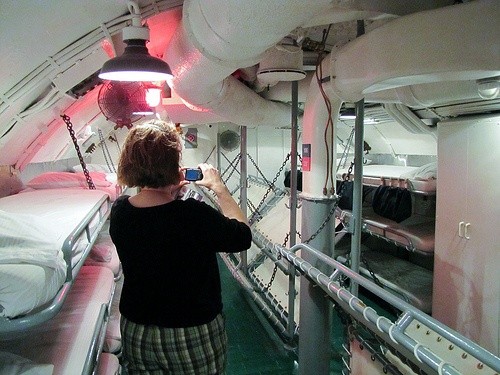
[336,165,436,257]
[0,162,122,375]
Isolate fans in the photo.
[98,79,149,130]
[219,130,241,152]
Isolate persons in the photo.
[109,118,252,375]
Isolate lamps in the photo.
[340,108,356,119]
[143,83,163,108]
[97,0,174,82]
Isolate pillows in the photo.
[26,172,112,190]
[410,162,437,180]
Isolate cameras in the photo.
[185,169,202,181]
